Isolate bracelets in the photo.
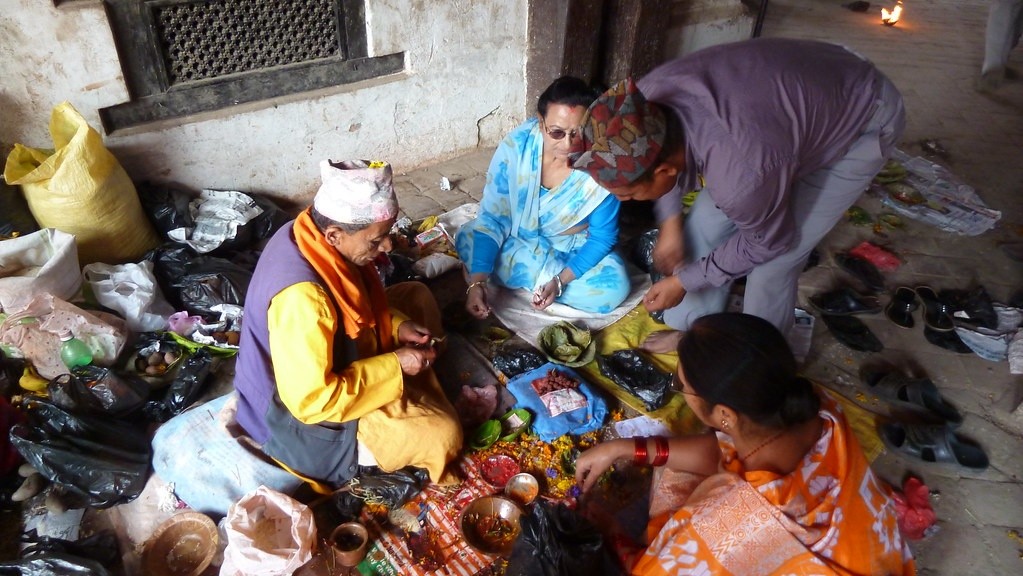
[466,281,487,294]
[632,435,647,467]
[553,274,563,297]
[648,434,670,467]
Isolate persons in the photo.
[589,36,907,354]
[231,156,465,487]
[574,310,923,575]
[455,71,630,318]
[977,2,1023,93]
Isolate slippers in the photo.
[876,419,989,473]
[833,252,884,290]
[916,285,954,331]
[807,285,881,316]
[883,287,921,330]
[858,359,963,430]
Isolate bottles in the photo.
[57,329,99,378]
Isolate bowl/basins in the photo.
[505,473,539,506]
[479,454,520,489]
[142,511,218,576]
[458,495,525,558]
[887,183,923,206]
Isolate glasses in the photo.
[541,119,576,140]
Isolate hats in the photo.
[566,77,665,190]
[314,158,399,224]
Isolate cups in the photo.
[329,522,368,567]
